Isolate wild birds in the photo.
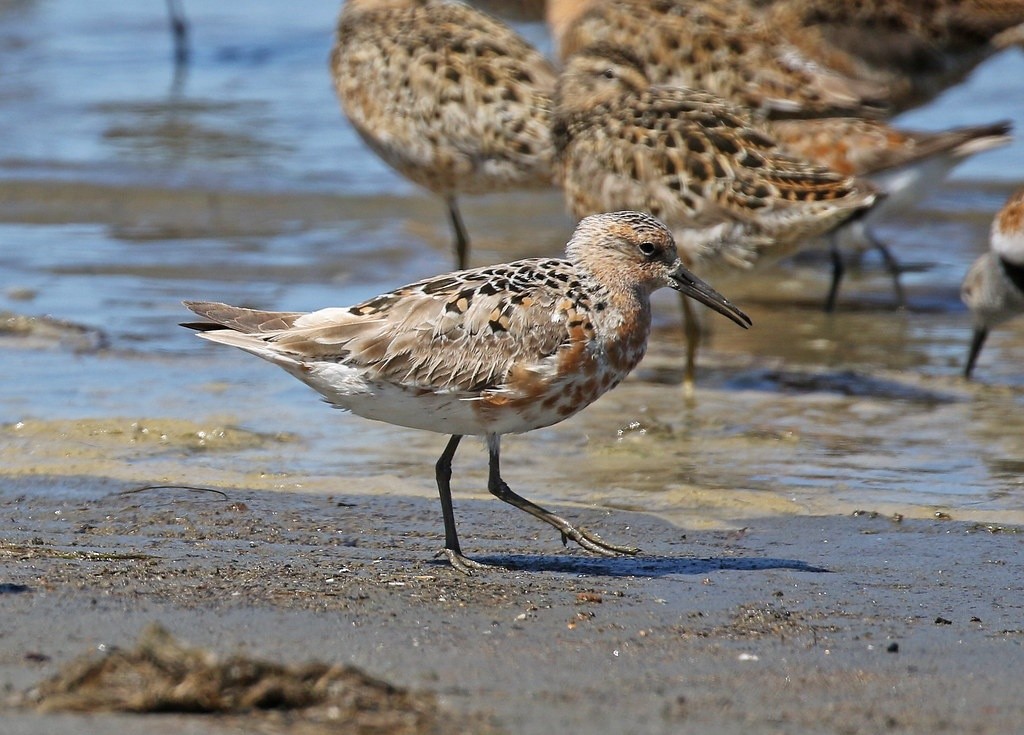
[177,0,1024,576]
